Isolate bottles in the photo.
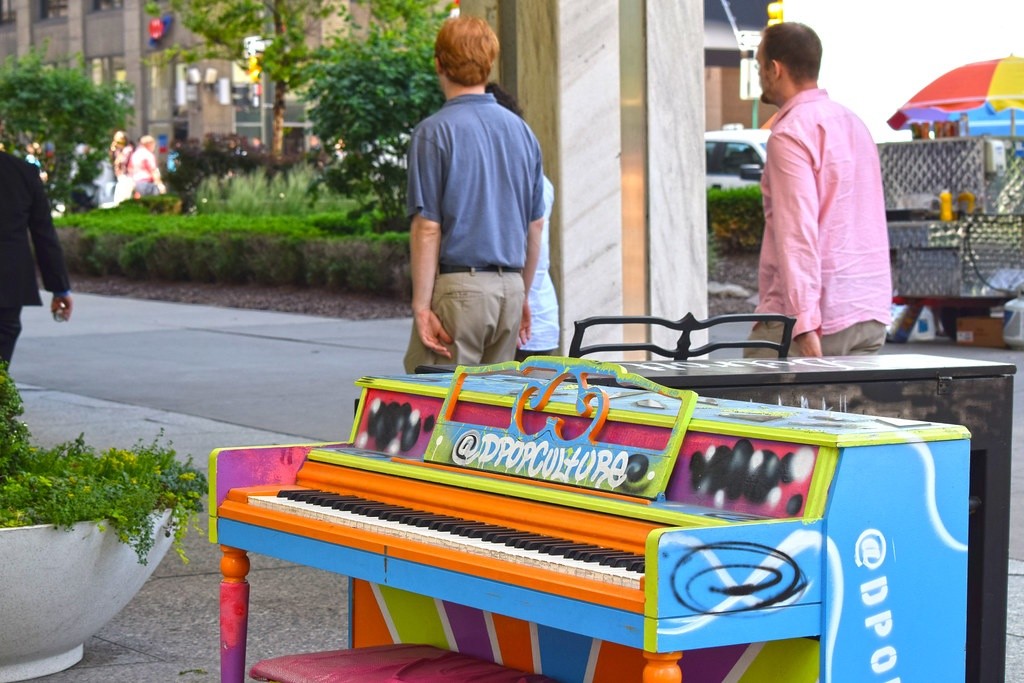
[959,112,969,138]
[939,185,954,221]
[53,299,67,323]
[957,184,974,214]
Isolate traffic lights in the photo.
[767,2,783,26]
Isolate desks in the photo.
[888,294,1018,345]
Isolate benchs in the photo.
[246,642,560,683]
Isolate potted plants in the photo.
[0,354,210,683]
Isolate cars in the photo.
[704,128,772,189]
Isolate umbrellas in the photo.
[887,54,1024,136]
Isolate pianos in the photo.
[203,350,975,683]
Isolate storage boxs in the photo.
[954,315,1008,352]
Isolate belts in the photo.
[438,259,522,274]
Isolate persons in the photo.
[743,22,892,359]
[403,14,559,375]
[0,150,73,371]
[0,131,348,209]
